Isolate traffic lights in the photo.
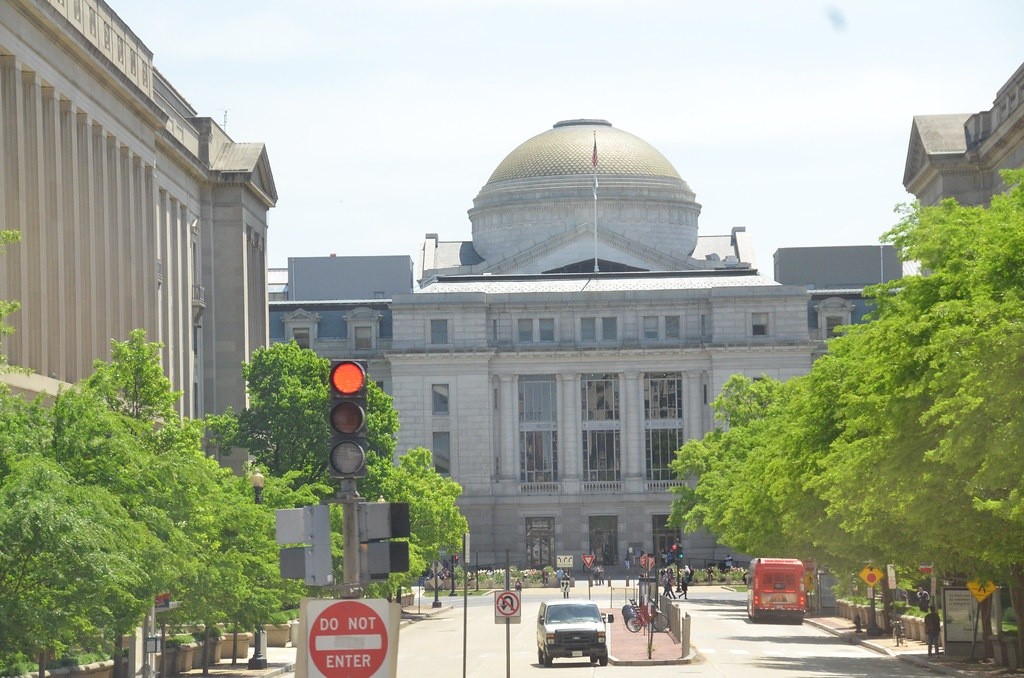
[672,542,683,564]
[330,358,367,479]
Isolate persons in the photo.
[924,604,940,657]
[916,585,930,612]
[514,539,748,599]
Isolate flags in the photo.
[591,149,598,201]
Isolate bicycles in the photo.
[622,595,669,632]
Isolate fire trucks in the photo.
[742,557,806,624]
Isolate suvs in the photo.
[536,599,614,667]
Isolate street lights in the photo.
[248,466,267,671]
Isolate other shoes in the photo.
[935,654,939,658]
[927,655,932,659]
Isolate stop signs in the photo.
[640,553,654,568]
[309,601,388,678]
